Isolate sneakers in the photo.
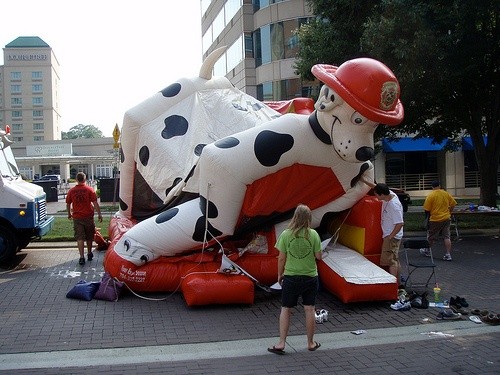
[315,308,328,323]
[419,248,430,256]
[442,255,452,260]
[390,288,500,325]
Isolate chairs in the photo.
[403,240,438,294]
[451,215,458,238]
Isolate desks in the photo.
[450,204,500,214]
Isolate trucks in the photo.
[0,141,54,267]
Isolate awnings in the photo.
[383,137,452,152]
[462,136,488,151]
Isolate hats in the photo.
[432,176,442,186]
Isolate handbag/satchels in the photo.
[66,279,101,302]
[95,272,123,302]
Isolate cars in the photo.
[390,188,408,213]
[33,175,60,185]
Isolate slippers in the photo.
[309,341,321,351]
[268,346,285,355]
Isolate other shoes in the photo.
[88,252,93,261]
[79,257,85,264]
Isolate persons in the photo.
[66,173,102,265]
[374,183,405,285]
[420,181,457,261]
[268,205,323,354]
